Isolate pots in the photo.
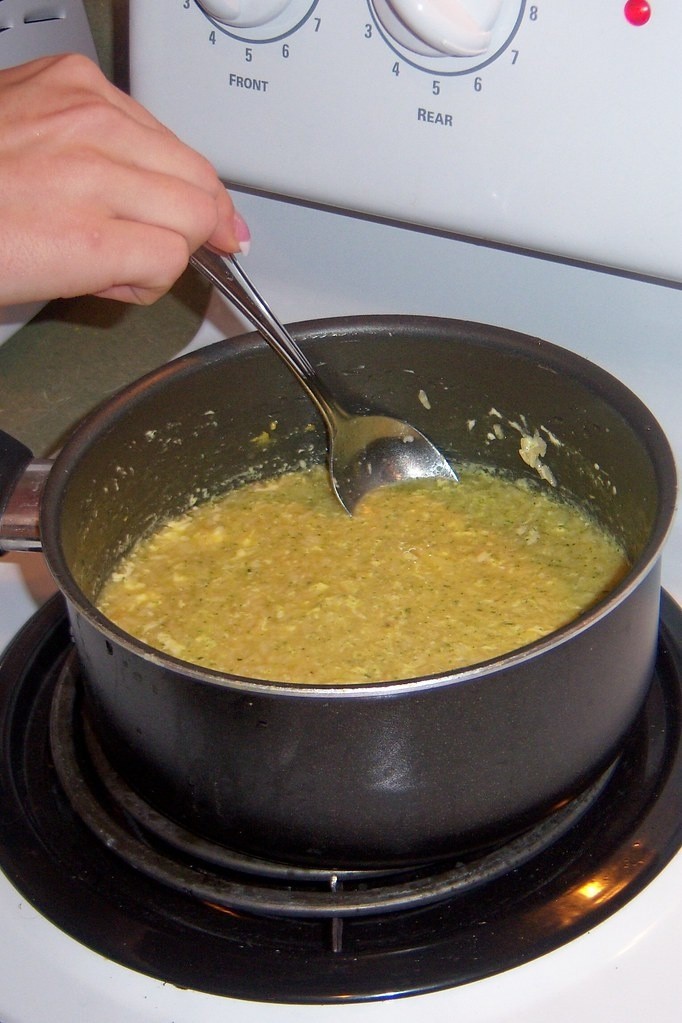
[0,314,677,849]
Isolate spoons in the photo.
[189,241,459,518]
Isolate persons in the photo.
[0,53,251,306]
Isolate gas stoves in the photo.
[0,0,682,1022]
